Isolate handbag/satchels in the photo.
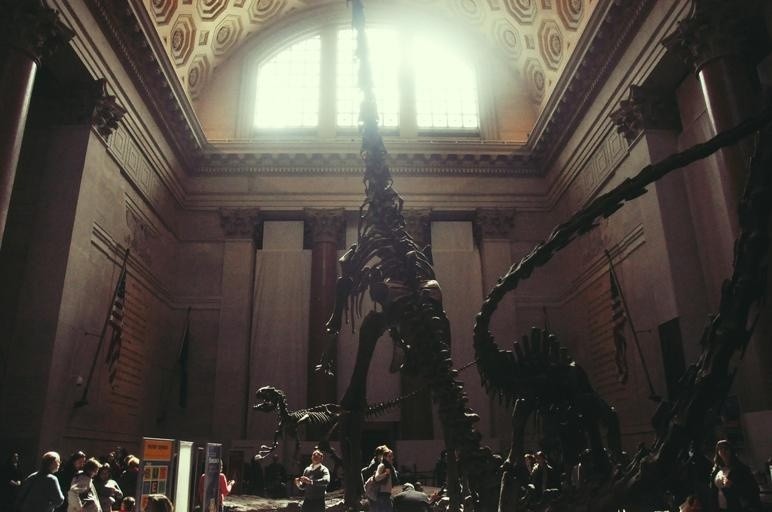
[362,475,378,502]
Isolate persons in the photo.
[1,447,141,512]
[145,494,174,512]
[361,441,644,510]
[300,450,330,512]
[709,440,763,511]
[199,458,235,512]
[679,493,707,512]
